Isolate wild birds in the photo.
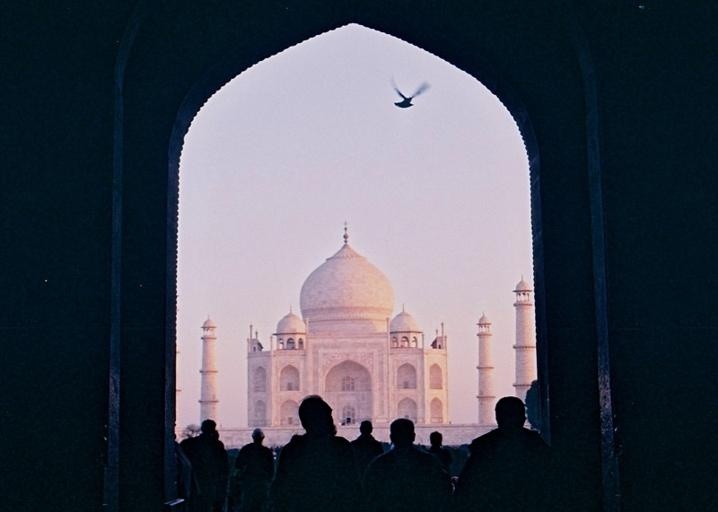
[389,73,429,108]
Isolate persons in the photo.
[350,420,381,467]
[268,395,361,512]
[427,431,451,467]
[452,396,557,510]
[179,419,230,511]
[362,417,452,511]
[235,427,274,511]
